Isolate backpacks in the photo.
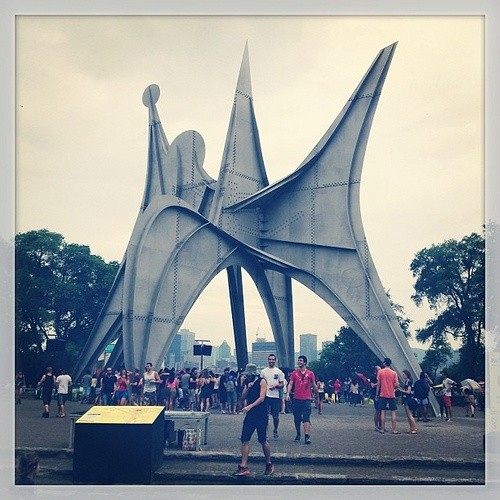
[225,377,235,393]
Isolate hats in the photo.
[241,364,260,376]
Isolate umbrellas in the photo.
[357,374,369,385]
[460,379,481,391]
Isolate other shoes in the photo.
[392,430,401,434]
[295,433,301,442]
[233,465,250,476]
[413,412,474,422]
[265,462,274,475]
[273,429,279,438]
[406,426,417,434]
[305,433,311,444]
[374,425,384,433]
[55,412,65,418]
[42,411,50,418]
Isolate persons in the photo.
[156,368,247,415]
[16,371,24,395]
[316,376,366,414]
[464,388,476,417]
[438,371,458,421]
[397,369,418,434]
[260,354,285,440]
[230,363,275,476]
[40,367,56,418]
[81,367,140,406]
[139,363,163,406]
[413,371,434,422]
[370,365,382,431]
[54,367,72,417]
[285,356,320,444]
[375,358,401,434]
